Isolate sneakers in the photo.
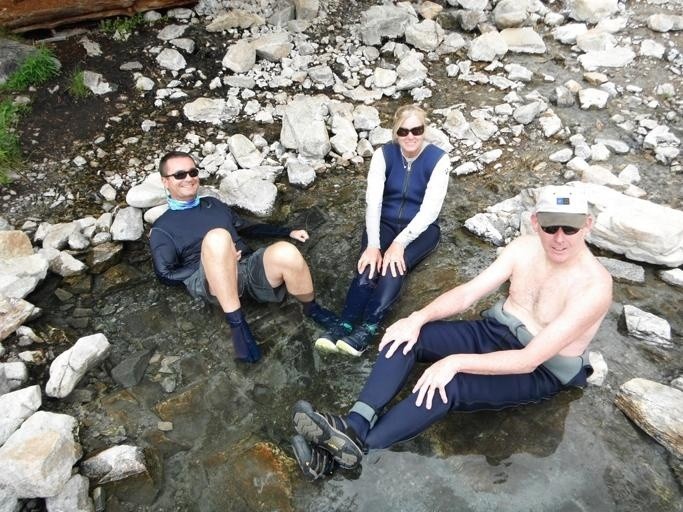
[293,435,338,484]
[335,323,374,359]
[291,398,365,472]
[313,315,354,355]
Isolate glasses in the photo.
[540,226,580,236]
[396,125,426,137]
[163,168,199,180]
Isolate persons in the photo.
[150,151,340,366]
[292,187,613,484]
[315,104,451,357]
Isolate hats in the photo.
[534,183,590,229]
